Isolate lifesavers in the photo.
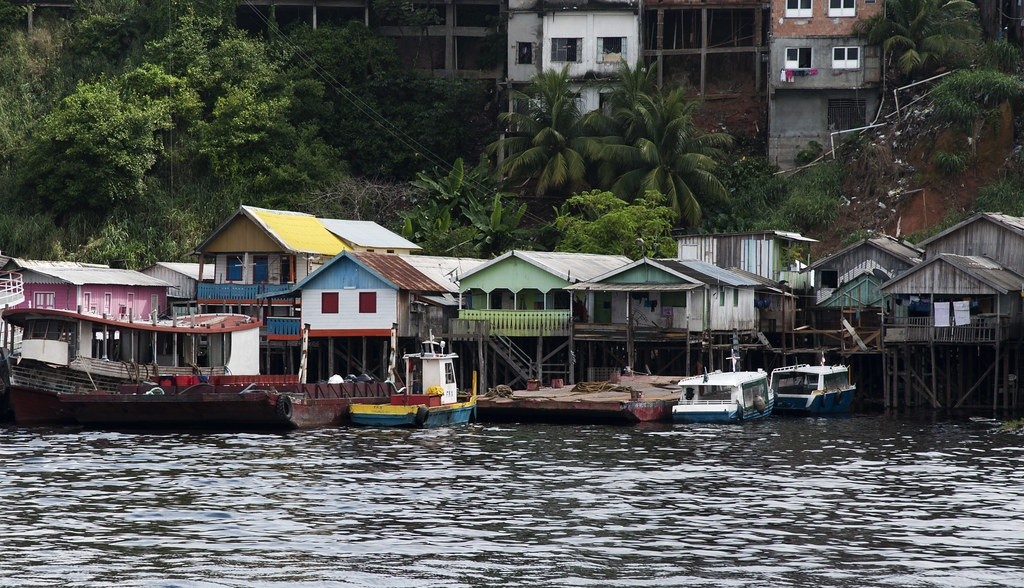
[415,406,430,424]
[275,395,294,419]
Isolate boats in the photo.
[671,350,775,424]
[769,350,857,416]
[0,302,262,426]
[347,328,474,429]
